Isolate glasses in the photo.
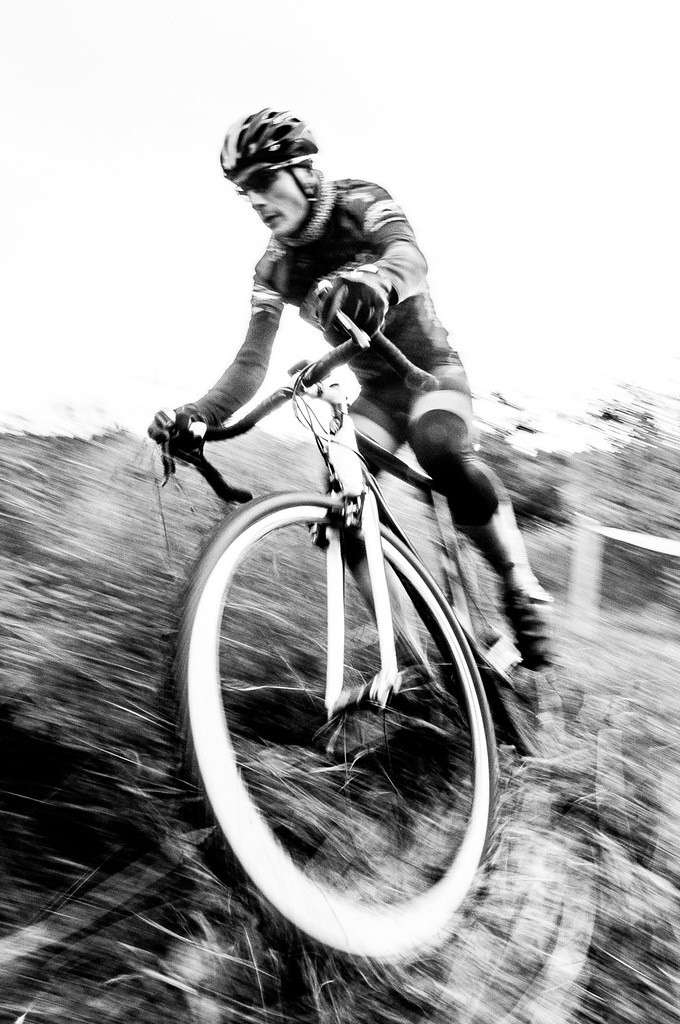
[235,168,279,194]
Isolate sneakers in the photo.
[503,593,558,667]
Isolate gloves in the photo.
[313,263,392,343]
[147,404,204,444]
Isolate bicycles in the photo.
[150,275,585,985]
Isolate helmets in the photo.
[220,107,318,184]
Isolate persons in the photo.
[145,107,565,670]
